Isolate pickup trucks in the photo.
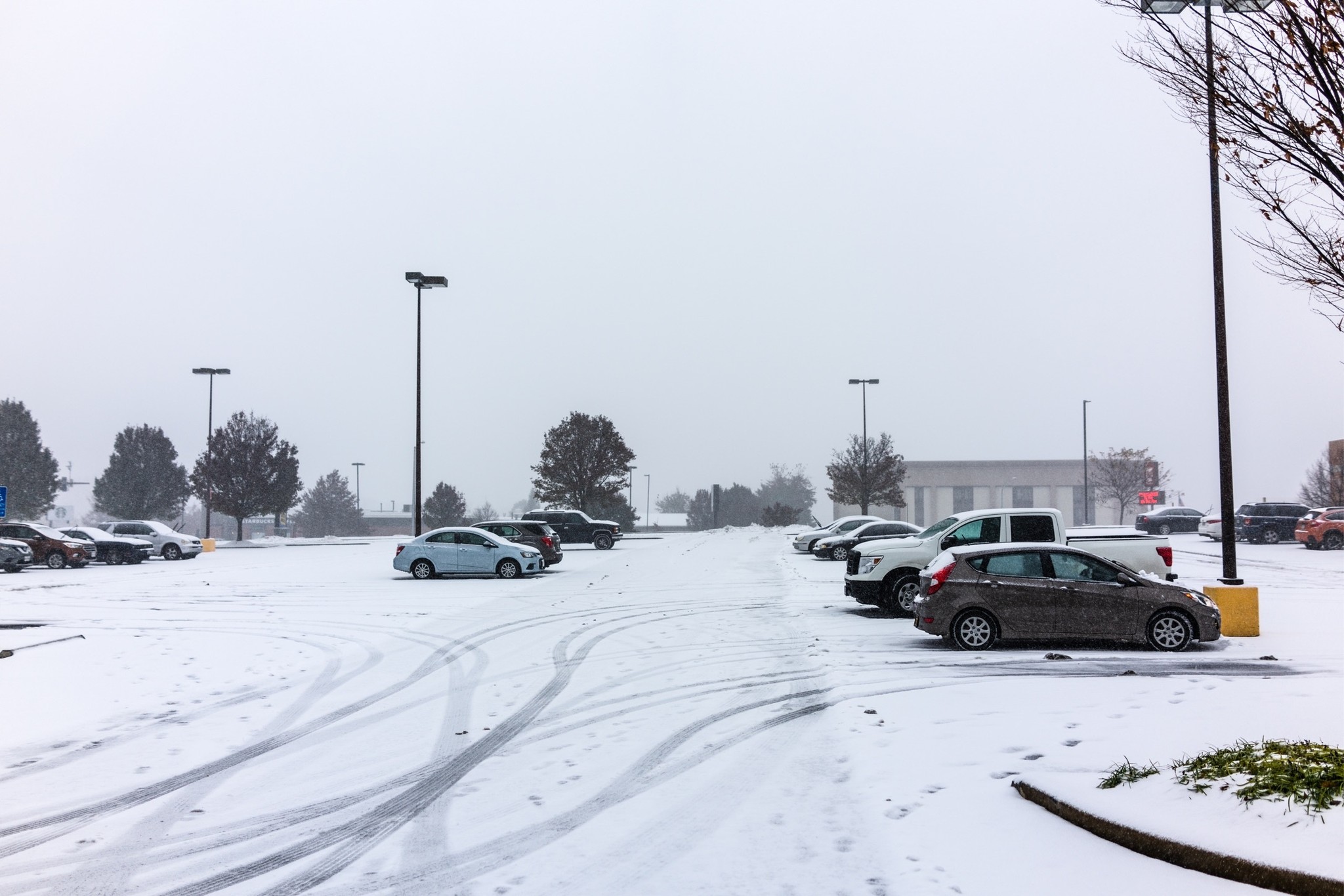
[844,508,1178,618]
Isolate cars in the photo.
[0,538,34,574]
[54,526,154,565]
[813,521,927,561]
[393,526,545,580]
[1294,506,1344,551]
[1198,510,1242,542]
[792,516,886,555]
[1135,506,1209,535]
[914,541,1221,653]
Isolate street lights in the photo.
[1083,399,1092,526]
[628,466,637,507]
[643,474,650,533]
[192,367,230,552]
[352,463,365,537]
[1140,0,1275,639]
[848,379,879,477]
[405,271,448,538]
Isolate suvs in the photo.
[97,520,203,561]
[469,520,563,569]
[1234,502,1314,545]
[521,509,623,550]
[0,521,97,570]
